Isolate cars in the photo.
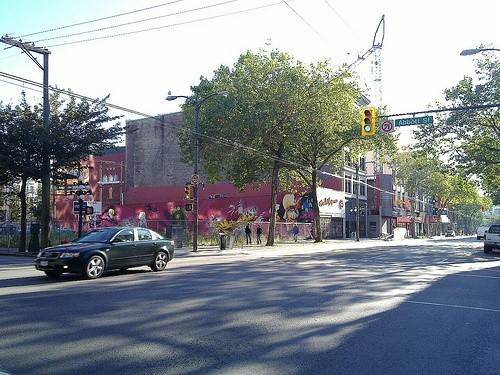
[476,227,489,240]
[34,226,175,279]
[483,224,500,254]
[447,230,455,237]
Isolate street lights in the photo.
[166,90,229,254]
[68,176,93,237]
[355,160,377,241]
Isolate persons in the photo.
[292,224,299,242]
[245,224,252,244]
[257,225,263,244]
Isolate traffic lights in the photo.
[362,106,376,138]
[85,207,94,214]
[184,185,190,201]
